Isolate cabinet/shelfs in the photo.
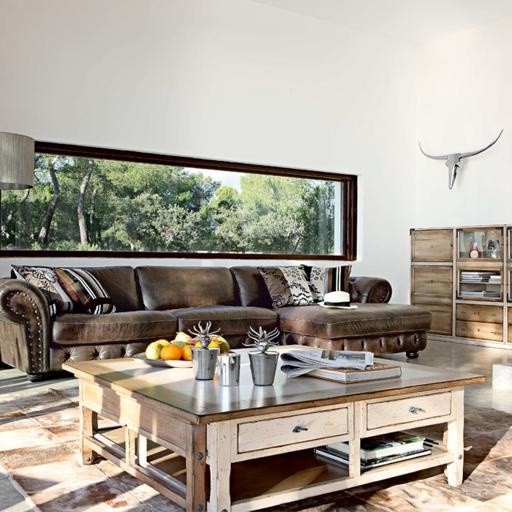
[410,224,512,352]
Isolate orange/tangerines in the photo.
[160,344,183,359]
[182,344,191,360]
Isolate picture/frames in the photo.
[0,136,358,262]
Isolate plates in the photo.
[133,350,238,368]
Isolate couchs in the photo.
[0,260,435,382]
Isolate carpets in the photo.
[0,384,511,511]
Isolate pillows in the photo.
[9,261,116,315]
[257,263,354,310]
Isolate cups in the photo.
[248,351,279,386]
[191,347,221,380]
[218,352,241,387]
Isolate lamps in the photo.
[0,131,36,191]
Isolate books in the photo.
[459,269,502,297]
[326,430,425,460]
[313,443,431,469]
[302,360,403,384]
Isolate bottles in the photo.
[469,241,482,258]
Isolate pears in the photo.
[145,341,162,360]
[174,331,192,343]
